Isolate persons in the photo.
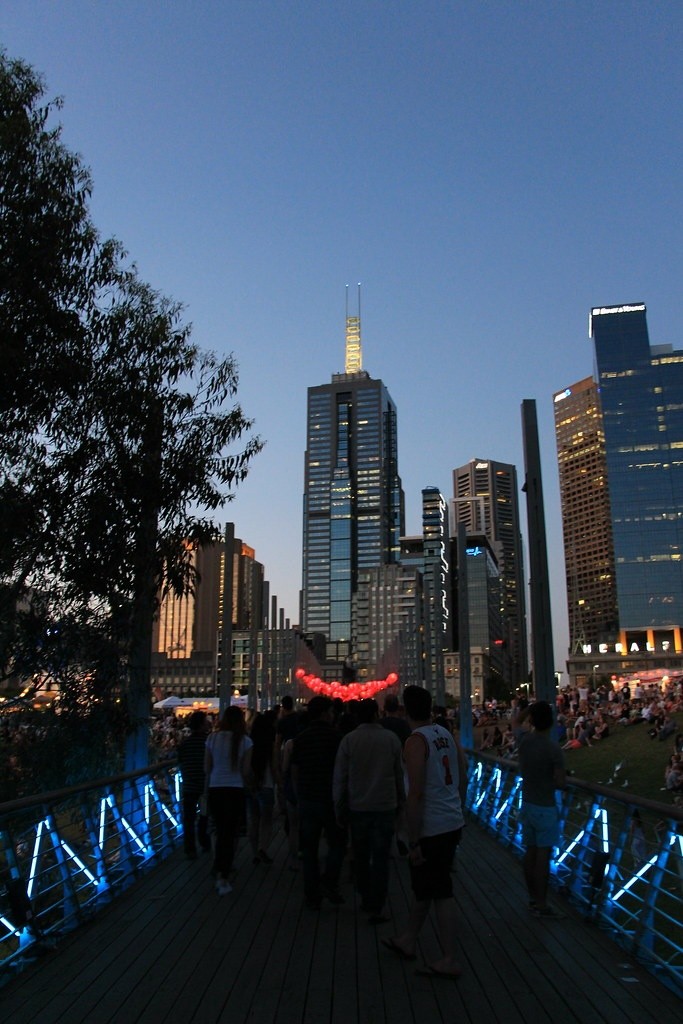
[331,697,405,922]
[178,693,465,908]
[444,676,683,759]
[379,684,468,980]
[204,704,259,897]
[517,700,568,920]
[663,733,683,792]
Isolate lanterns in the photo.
[296,669,398,701]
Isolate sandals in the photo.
[528,901,536,911]
[533,906,566,918]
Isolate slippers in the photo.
[381,937,417,960]
[416,964,460,979]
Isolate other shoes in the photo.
[186,852,199,861]
[259,849,268,862]
[303,895,322,908]
[215,881,220,890]
[253,856,260,865]
[321,885,347,905]
[369,908,384,923]
[219,885,232,896]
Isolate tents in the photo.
[154,695,193,709]
